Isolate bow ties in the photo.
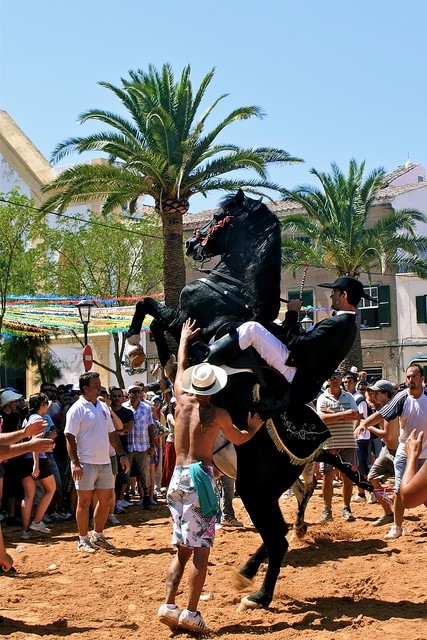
[332,311,337,316]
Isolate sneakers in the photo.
[220,515,243,527]
[60,512,72,519]
[46,511,64,521]
[78,540,96,553]
[317,511,333,523]
[90,535,115,550]
[368,494,375,504]
[384,525,402,540]
[350,494,367,502]
[178,609,205,635]
[30,521,51,533]
[125,500,134,507]
[157,604,181,629]
[108,514,120,525]
[7,517,21,526]
[372,513,393,526]
[150,498,158,505]
[115,504,126,514]
[142,497,152,509]
[21,529,41,540]
[341,507,355,521]
[121,501,129,508]
[43,513,52,523]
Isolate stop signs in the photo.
[83,345,93,371]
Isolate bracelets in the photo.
[119,454,126,457]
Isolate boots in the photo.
[187,328,243,366]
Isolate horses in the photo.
[122,188,375,611]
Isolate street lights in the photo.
[75,298,95,372]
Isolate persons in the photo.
[19,382,78,428]
[317,370,359,522]
[344,374,377,504]
[111,388,135,514]
[0,420,56,463]
[366,380,399,527]
[34,414,63,529]
[400,428,427,509]
[91,396,124,527]
[202,276,382,405]
[0,389,23,527]
[121,384,156,510]
[141,382,177,498]
[64,372,131,553]
[364,390,382,466]
[21,393,57,540]
[354,363,427,540]
[355,371,407,399]
[158,316,265,633]
[63,459,77,521]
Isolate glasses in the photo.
[329,378,340,382]
[51,390,57,394]
[111,394,122,397]
[130,391,140,394]
[42,388,52,392]
[65,398,73,402]
[59,392,65,396]
[42,401,49,406]
[70,392,81,396]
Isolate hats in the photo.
[151,395,162,402]
[4,387,19,393]
[127,385,142,394]
[331,371,342,377]
[367,380,393,395]
[0,390,23,407]
[171,397,176,403]
[318,277,380,303]
[182,363,228,395]
[72,382,80,391]
